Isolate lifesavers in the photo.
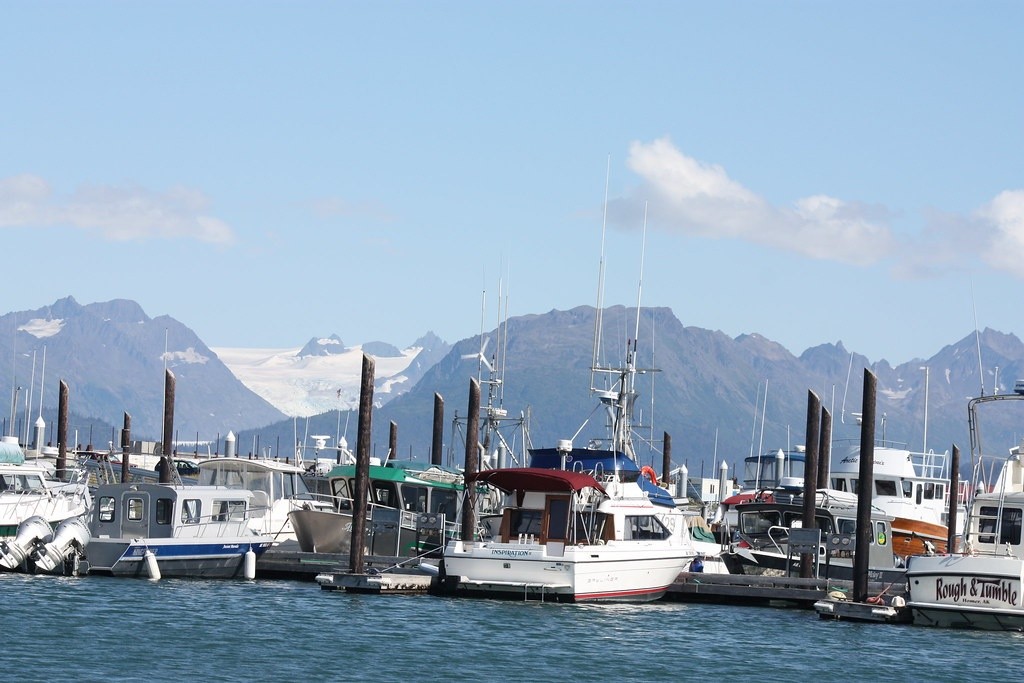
[867,597,885,604]
[641,466,656,486]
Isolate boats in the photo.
[0,154,1024,630]
